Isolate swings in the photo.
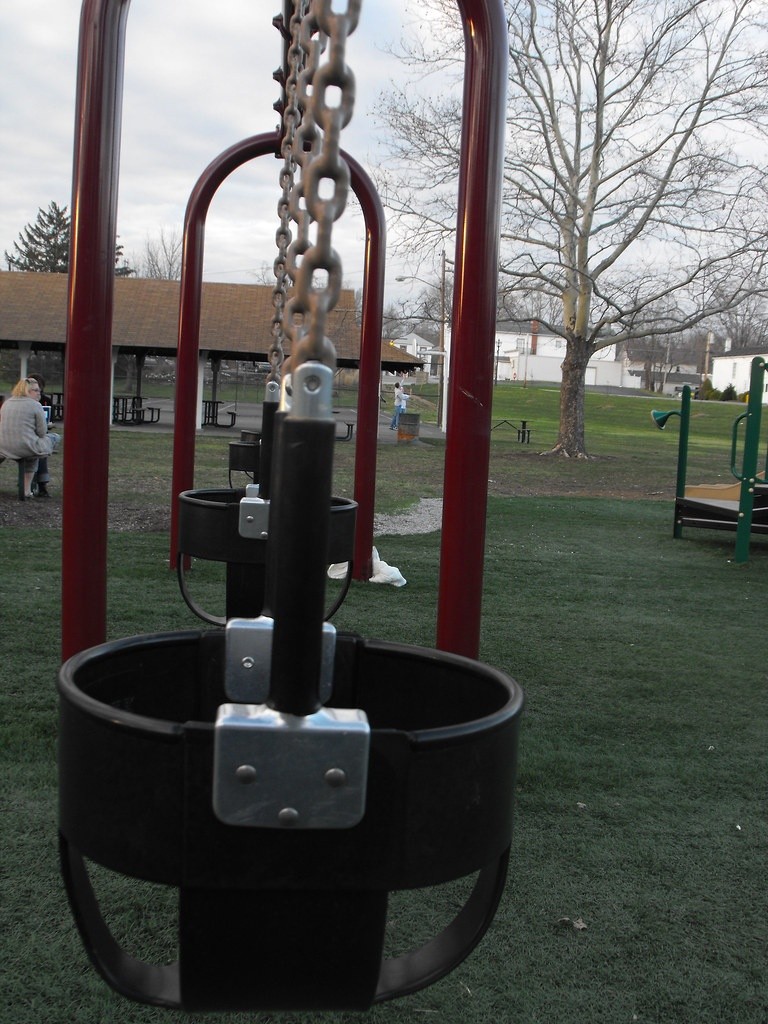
[49,1,532,1018]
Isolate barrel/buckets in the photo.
[398,413,420,443]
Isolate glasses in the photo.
[31,388,42,393]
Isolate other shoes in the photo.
[394,427,398,431]
[391,426,394,430]
[24,491,33,500]
[38,489,49,497]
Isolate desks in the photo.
[45,392,64,404]
[491,419,534,444]
[113,395,148,426]
[201,400,224,426]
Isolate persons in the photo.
[389,382,411,430]
[0,378,62,498]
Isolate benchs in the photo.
[335,421,355,442]
[217,411,236,428]
[144,406,162,424]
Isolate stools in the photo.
[18,455,49,501]
[50,404,64,423]
[132,408,145,424]
[518,428,536,444]
